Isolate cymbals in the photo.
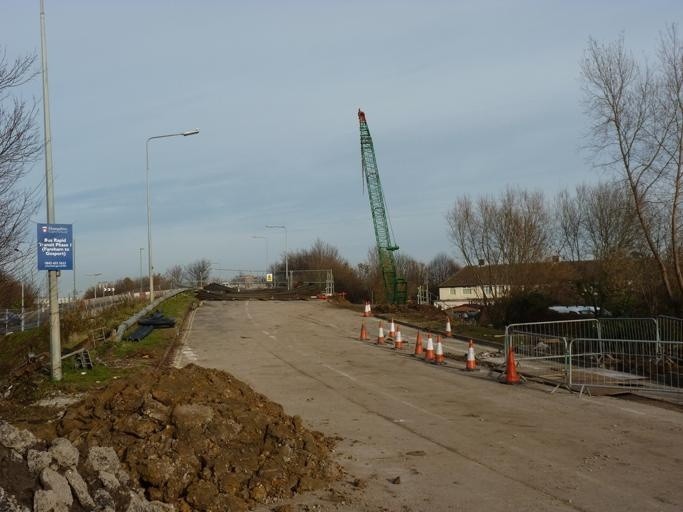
[358,108,406,303]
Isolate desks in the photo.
[146,128,201,303]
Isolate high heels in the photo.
[360,301,402,350]
[445,317,452,337]
[415,329,447,365]
[503,347,521,384]
[463,339,480,371]
